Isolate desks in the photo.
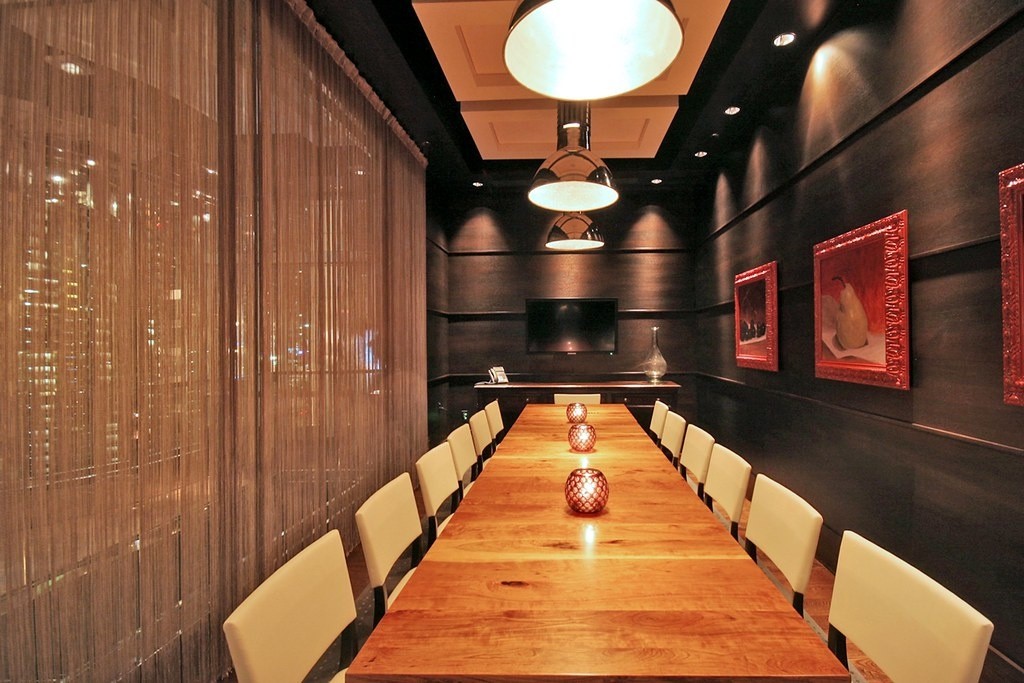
[345,404,852,683]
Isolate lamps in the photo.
[528,121,620,212]
[501,0,685,102]
[545,211,605,251]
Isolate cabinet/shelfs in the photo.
[474,382,682,462]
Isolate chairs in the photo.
[553,393,601,405]
[223,399,995,683]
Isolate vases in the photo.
[642,326,667,384]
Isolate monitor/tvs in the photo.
[524,297,619,356]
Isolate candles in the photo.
[564,467,609,514]
[566,403,588,424]
[568,424,597,452]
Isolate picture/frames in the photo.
[813,208,909,390]
[998,162,1024,406]
[735,260,779,372]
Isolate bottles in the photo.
[643,326,668,384]
